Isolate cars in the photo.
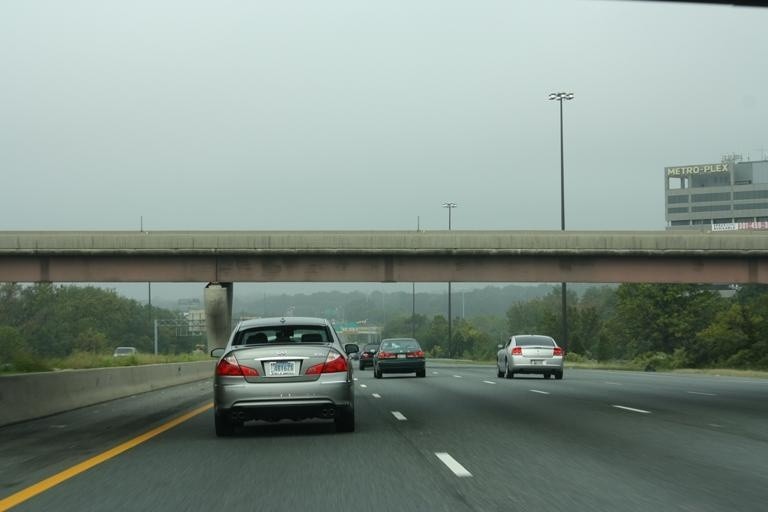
[497,335,564,379]
[359,337,425,378]
[113,347,137,356]
[211,316,359,435]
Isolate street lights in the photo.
[441,200,457,352]
[548,91,574,351]
